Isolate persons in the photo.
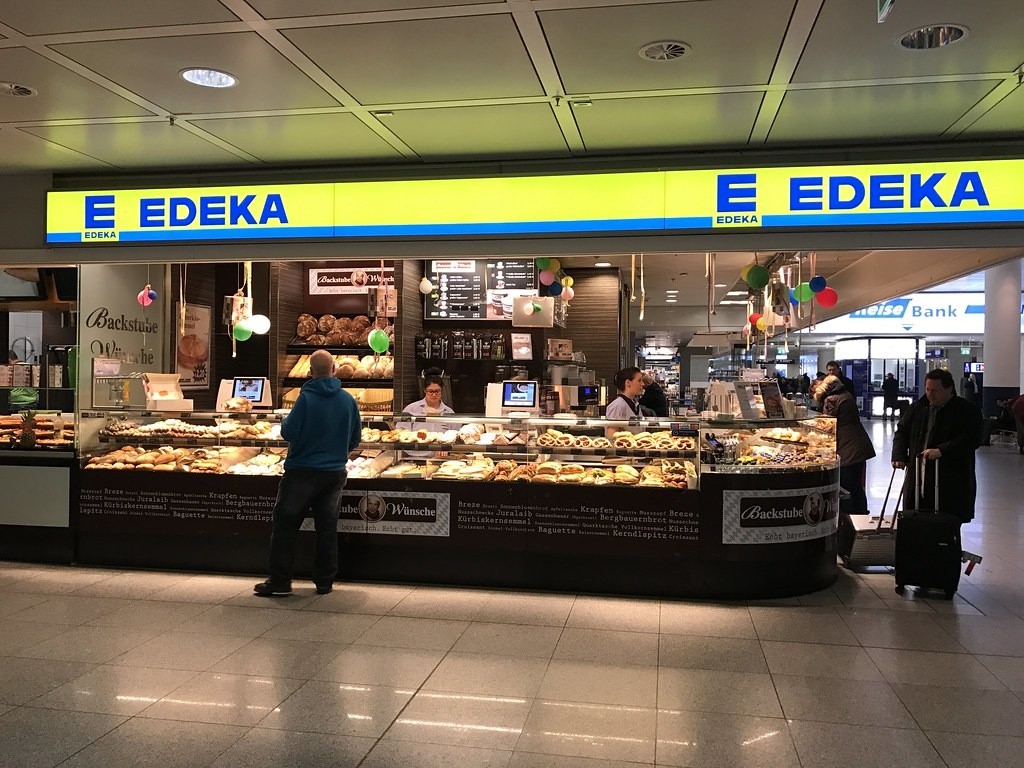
[647,370,665,384]
[606,366,645,421]
[7,350,38,413]
[964,373,983,407]
[764,370,810,399]
[403,368,461,459]
[892,369,984,593]
[882,373,899,421]
[807,360,876,515]
[639,371,670,417]
[252,349,362,598]
[980,394,1017,447]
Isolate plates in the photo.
[496,285,505,289]
[495,276,504,279]
[496,266,504,269]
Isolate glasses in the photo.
[425,389,441,395]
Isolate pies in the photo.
[0,416,74,445]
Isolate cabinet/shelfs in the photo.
[81,408,700,489]
[283,313,395,411]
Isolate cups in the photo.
[498,271,502,277]
[498,262,502,267]
[498,280,505,287]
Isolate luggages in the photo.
[895,452,962,600]
[836,460,912,569]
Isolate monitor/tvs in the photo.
[231,376,266,401]
[502,381,539,411]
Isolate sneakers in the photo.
[316,585,332,594]
[254,580,293,596]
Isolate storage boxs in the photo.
[140,373,193,411]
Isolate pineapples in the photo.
[20,409,36,449]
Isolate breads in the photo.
[344,424,538,482]
[288,355,395,380]
[738,418,838,461]
[535,428,698,450]
[82,418,285,475]
[297,313,395,346]
[531,462,662,487]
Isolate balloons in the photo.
[756,316,768,330]
[419,277,435,294]
[233,313,271,340]
[137,288,157,308]
[789,287,798,306]
[749,313,763,325]
[368,328,390,354]
[815,287,838,307]
[536,257,562,295]
[740,261,769,289]
[561,275,574,300]
[793,282,815,302]
[809,275,826,293]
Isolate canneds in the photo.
[491,292,537,319]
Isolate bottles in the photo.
[54,412,64,440]
